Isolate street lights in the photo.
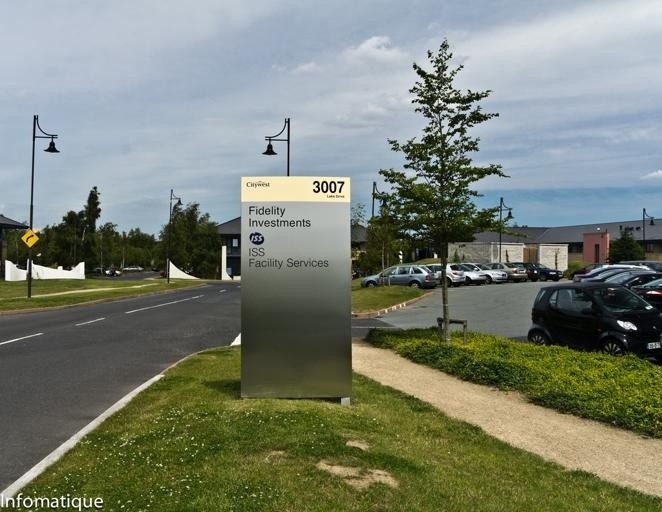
[27,113,63,302]
[372,181,389,218]
[262,118,293,177]
[168,188,183,284]
[499,197,515,263]
[642,208,655,256]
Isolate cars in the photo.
[96,267,121,276]
[123,265,144,272]
[527,261,662,365]
[361,255,563,288]
